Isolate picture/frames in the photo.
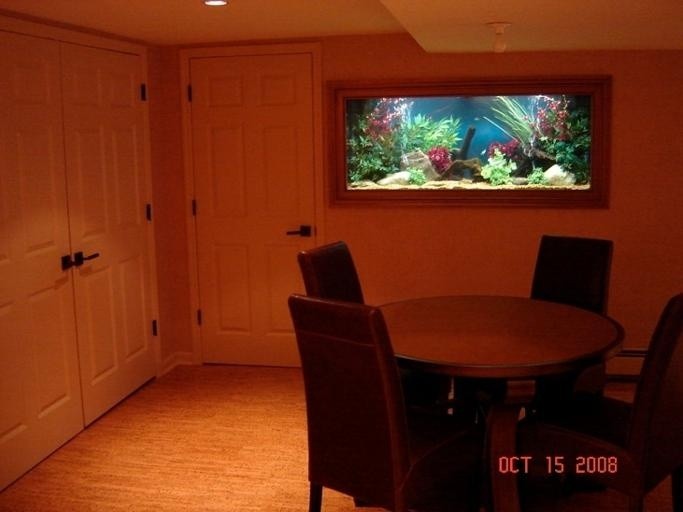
[324,76,611,209]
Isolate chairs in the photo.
[515,293,683,511]
[299,241,451,422]
[288,294,480,512]
[530,234,614,392]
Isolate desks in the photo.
[377,296,625,407]
